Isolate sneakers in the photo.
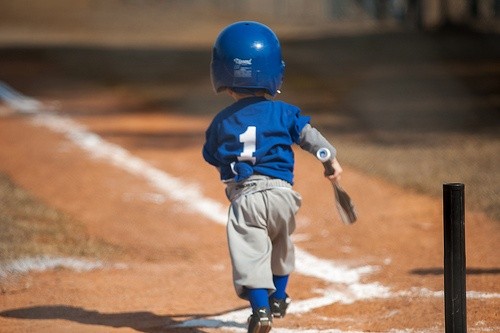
[247,307,272,333]
[269,291,290,318]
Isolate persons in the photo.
[202,21,343,333]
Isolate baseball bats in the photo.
[317,148,357,225]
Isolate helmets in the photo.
[210,21,287,97]
